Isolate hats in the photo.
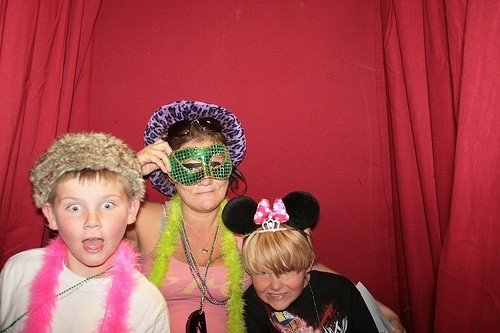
[143,100,245,196]
[31,131,145,209]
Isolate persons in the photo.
[1,130,170,332]
[219,185,380,333]
[125,97,407,332]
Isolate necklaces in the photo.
[147,192,247,332]
[1,229,139,332]
[282,272,323,333]
